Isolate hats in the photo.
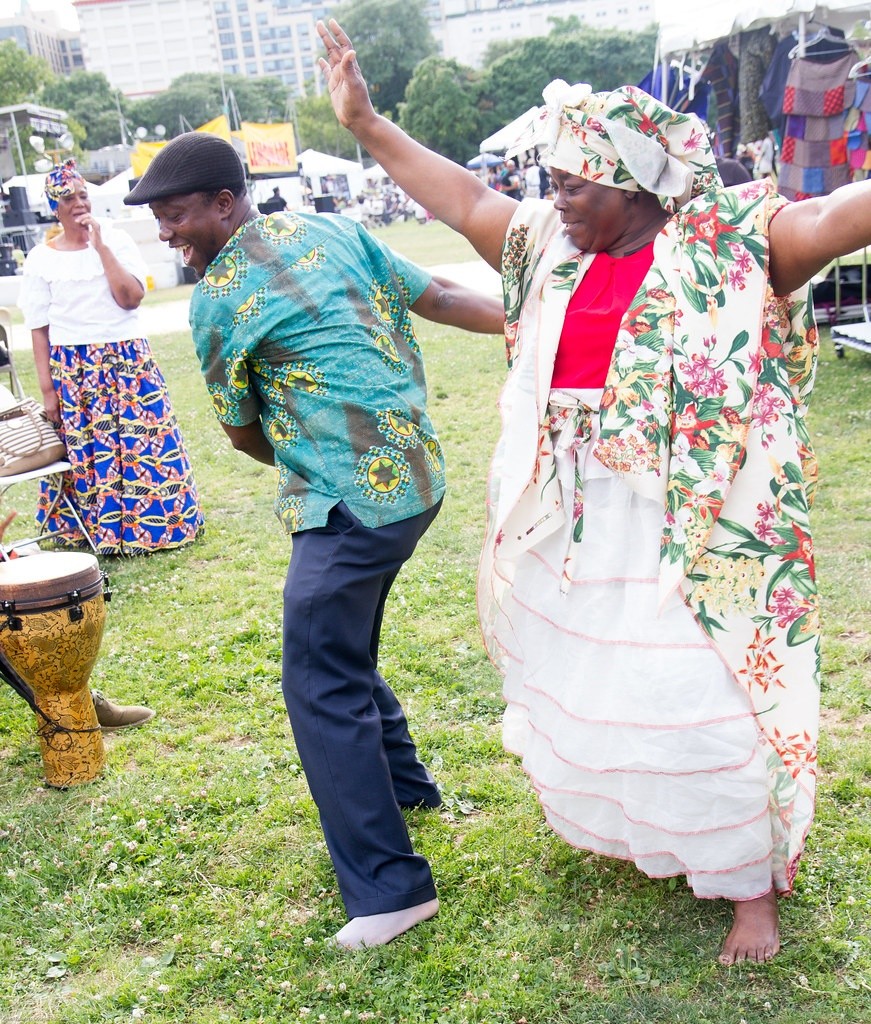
[124,132,244,205]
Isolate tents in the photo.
[3,147,389,291]
[658,0,871,106]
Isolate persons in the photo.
[0,652,155,730]
[315,17,871,964]
[267,187,292,211]
[20,156,206,554]
[698,118,751,189]
[124,131,508,950]
[314,159,552,225]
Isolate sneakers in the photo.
[90,688,156,730]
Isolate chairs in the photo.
[0,306,97,560]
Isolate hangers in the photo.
[669,52,711,102]
[847,36,871,79]
[769,0,852,60]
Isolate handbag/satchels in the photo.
[0,397,67,477]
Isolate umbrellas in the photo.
[469,155,504,171]
[359,160,392,178]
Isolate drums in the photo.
[0,550,114,790]
